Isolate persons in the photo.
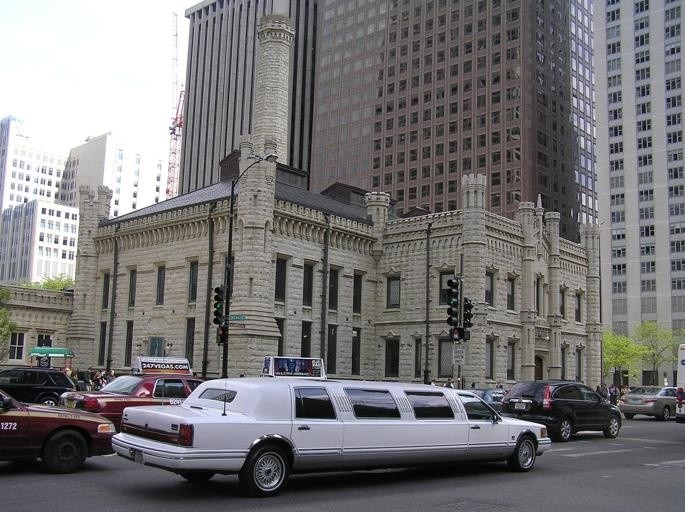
[430,379,436,387]
[595,382,630,406]
[443,378,454,388]
[495,379,503,388]
[63,367,117,392]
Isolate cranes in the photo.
[165,11,185,198]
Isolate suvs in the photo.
[0,369,76,407]
[501,380,622,441]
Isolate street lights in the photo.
[422,219,437,384]
[220,153,279,377]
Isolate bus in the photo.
[675,343,685,423]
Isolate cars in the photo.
[112,376,552,497]
[471,388,506,413]
[59,357,208,432]
[0,385,117,473]
[618,386,676,420]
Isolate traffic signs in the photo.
[229,315,245,320]
[229,322,245,329]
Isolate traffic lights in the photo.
[447,279,459,327]
[465,299,473,328]
[214,287,224,324]
[451,328,464,339]
[217,327,225,344]
